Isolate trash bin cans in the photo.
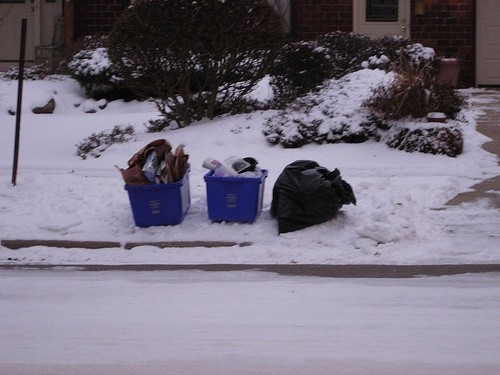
[202,157,269,224]
[123,138,191,227]
